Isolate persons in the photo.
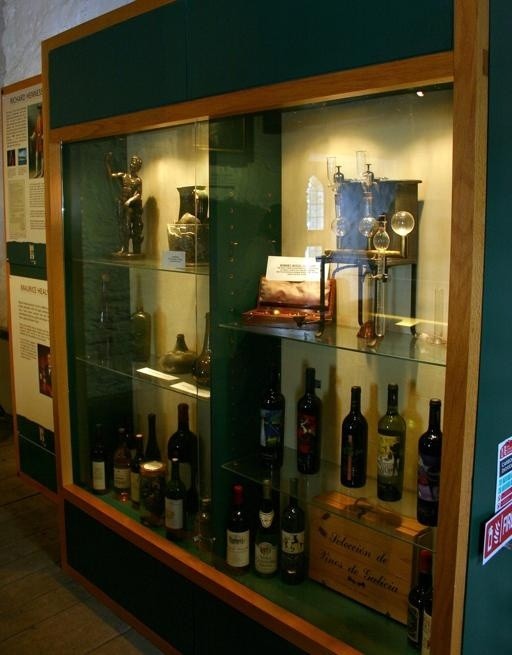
[38,354,54,397]
[29,103,49,178]
[102,150,144,256]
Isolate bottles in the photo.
[376,248,386,338]
[86,403,202,543]
[337,382,369,490]
[434,286,445,345]
[413,395,445,529]
[251,369,285,467]
[373,382,408,502]
[291,367,326,474]
[219,473,305,580]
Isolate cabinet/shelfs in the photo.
[43,0,512,654]
